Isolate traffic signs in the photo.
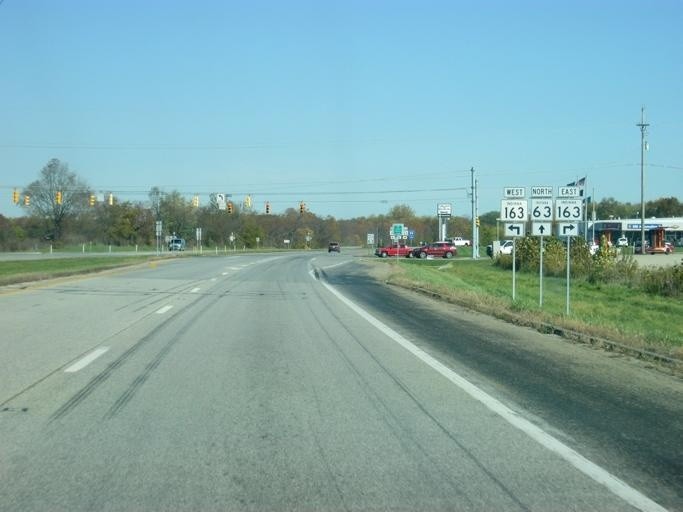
[389,223,414,240]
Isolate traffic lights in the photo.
[245,196,250,208]
[55,191,62,204]
[300,204,304,214]
[228,203,232,214]
[265,204,271,214]
[193,195,198,208]
[90,195,95,207]
[13,191,30,205]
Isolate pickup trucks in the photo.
[451,237,470,247]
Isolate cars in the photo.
[486,239,514,256]
[616,237,628,247]
[375,243,414,258]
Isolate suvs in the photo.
[169,239,185,251]
[416,242,457,259]
[328,242,340,252]
